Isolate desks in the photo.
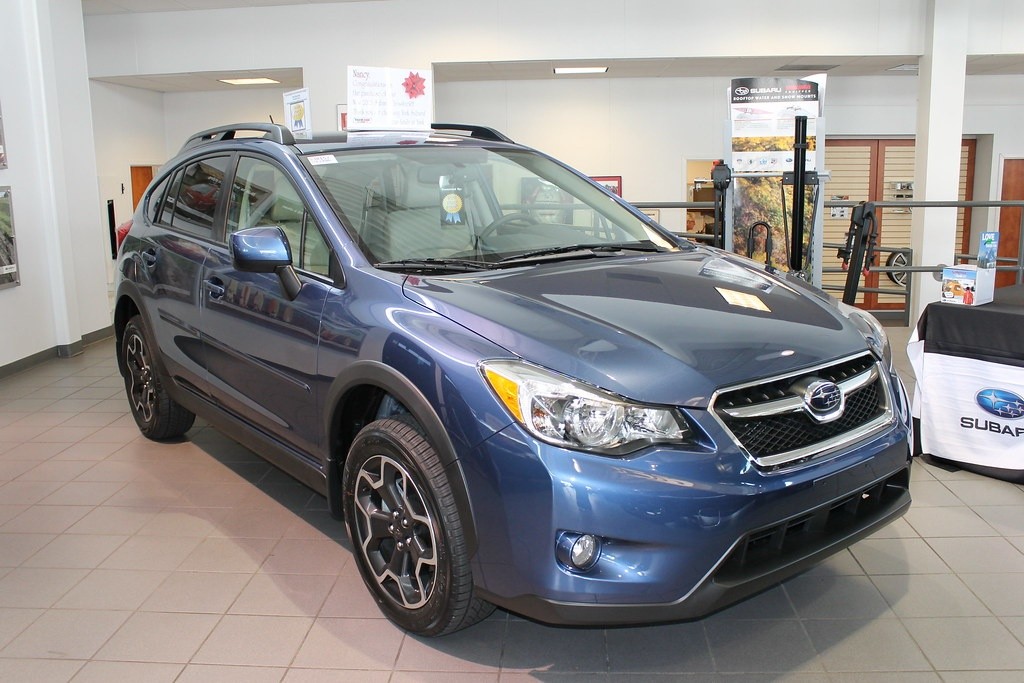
[905,283,1023,483]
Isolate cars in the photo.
[160,234,221,269]
[185,183,237,216]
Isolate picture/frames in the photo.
[639,208,659,223]
[520,176,572,224]
[0,185,20,290]
[0,98,7,168]
[587,175,622,198]
[336,103,346,132]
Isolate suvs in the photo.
[113,117,913,638]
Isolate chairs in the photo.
[256,177,330,281]
[382,164,473,260]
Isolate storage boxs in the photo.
[940,231,999,306]
